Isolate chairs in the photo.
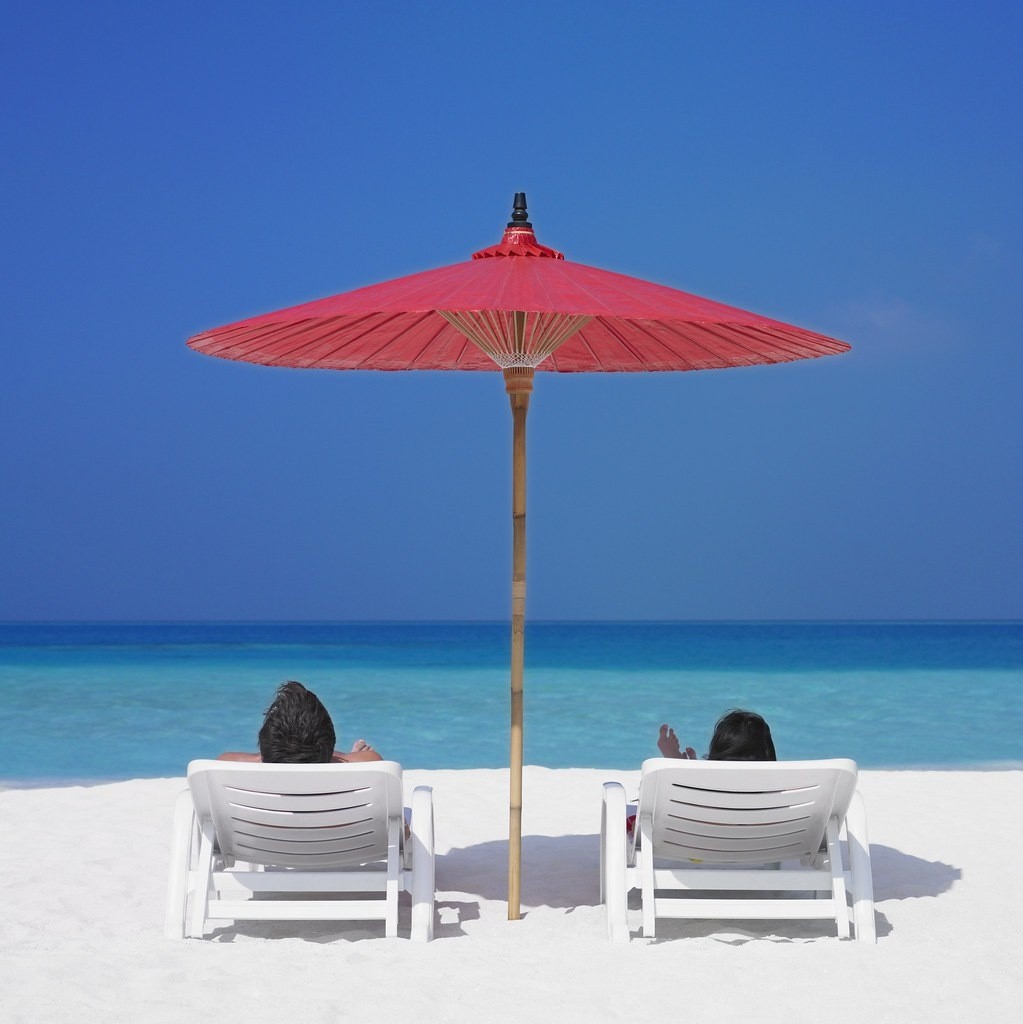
[598,758,877,949]
[180,760,436,944]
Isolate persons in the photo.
[217,680,407,864]
[628,708,790,860]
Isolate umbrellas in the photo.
[183,190,852,924]
